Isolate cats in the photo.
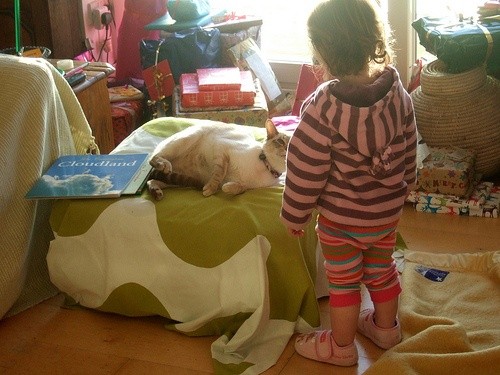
[147,120,292,202]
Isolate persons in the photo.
[279,0,418,368]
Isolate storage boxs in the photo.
[173,79,268,129]
[416,149,480,195]
[179,66,256,107]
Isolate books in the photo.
[24,153,157,199]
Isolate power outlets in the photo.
[94,6,109,29]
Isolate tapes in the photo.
[57,58,74,70]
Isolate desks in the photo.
[45,59,116,154]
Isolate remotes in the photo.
[66,73,86,88]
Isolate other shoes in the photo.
[358,308,402,349]
[295,330,359,366]
